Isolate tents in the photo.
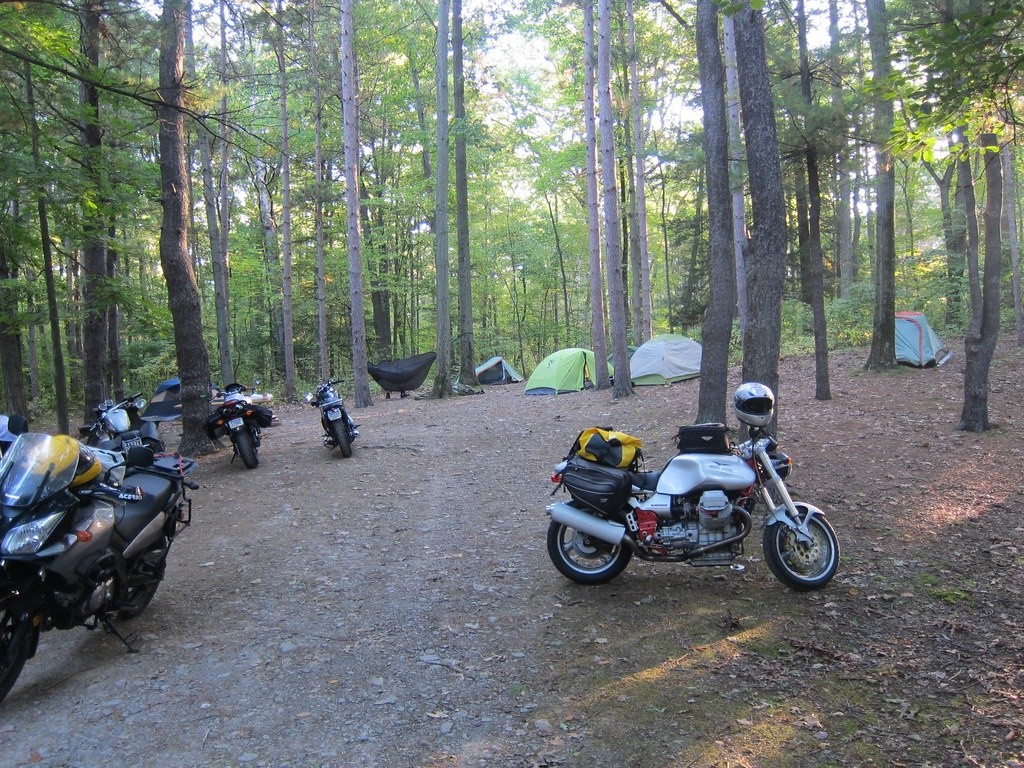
[140,376,219,421]
[475,356,523,385]
[629,333,702,386]
[894,310,948,368]
[606,346,639,367]
[524,348,614,395]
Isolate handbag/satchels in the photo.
[139,422,166,454]
[244,404,272,428]
[202,411,228,440]
[570,428,641,469]
[562,459,632,514]
[671,422,735,455]
[96,441,119,454]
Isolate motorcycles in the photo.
[79,393,155,465]
[304,375,361,459]
[203,377,272,469]
[1,414,200,703]
[545,422,842,595]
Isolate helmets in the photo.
[23,434,101,487]
[733,382,774,426]
